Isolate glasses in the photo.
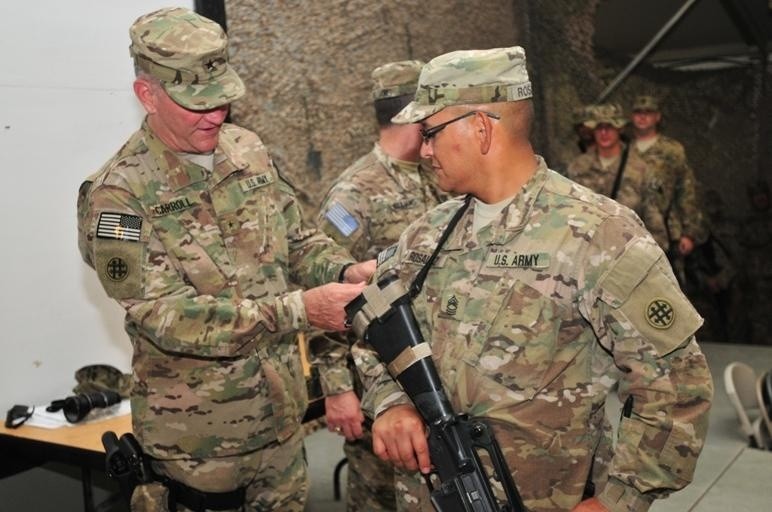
[418,108,500,145]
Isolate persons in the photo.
[564,99,631,203]
[739,180,770,345]
[301,59,463,512]
[349,43,713,512]
[75,5,379,511]
[698,186,751,345]
[614,90,703,299]
[538,103,593,174]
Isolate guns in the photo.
[100,430,154,511]
[344,274,524,507]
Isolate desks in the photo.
[0,346,327,510]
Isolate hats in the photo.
[128,6,246,114]
[571,103,632,130]
[369,45,533,126]
[632,96,661,112]
